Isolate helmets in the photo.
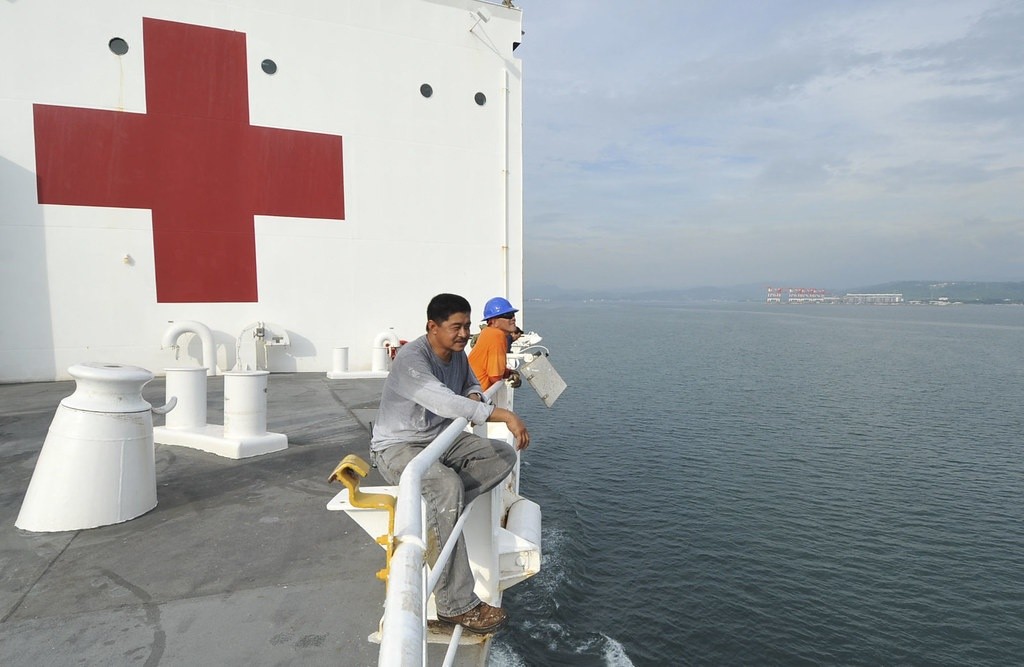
[481,297,519,321]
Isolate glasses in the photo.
[497,312,514,319]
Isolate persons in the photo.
[506,327,523,352]
[467,297,522,392]
[371,294,529,632]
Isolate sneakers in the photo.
[437,601,509,634]
